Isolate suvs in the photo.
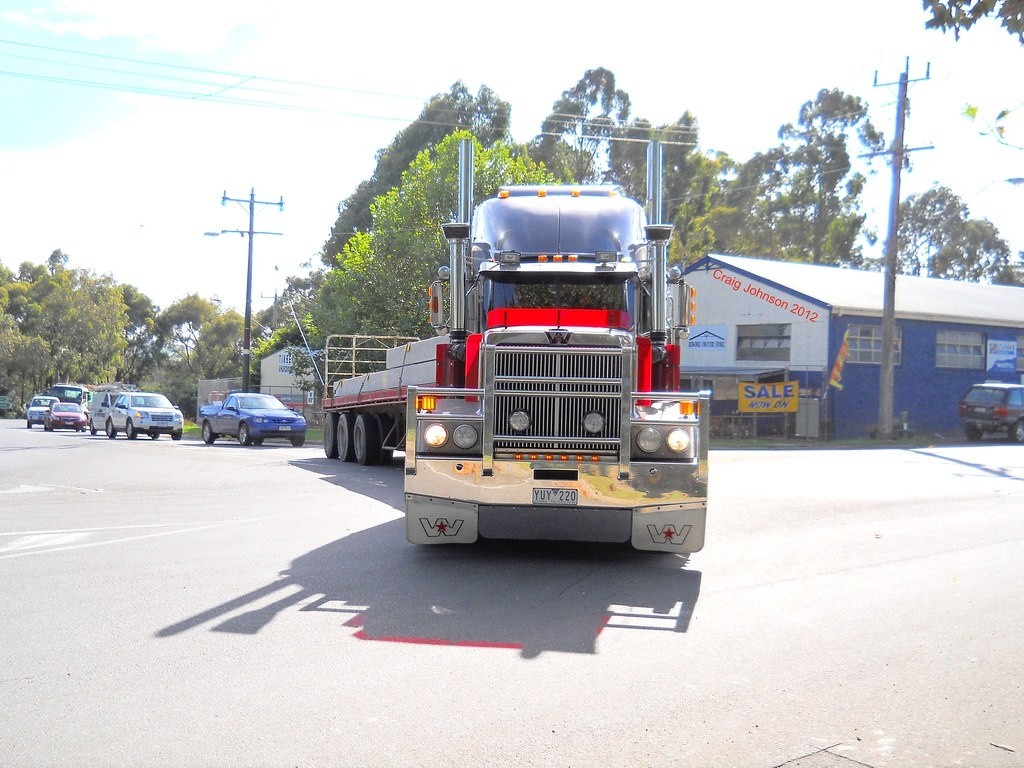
[960,383,1024,444]
[89,392,160,440]
[106,393,184,440]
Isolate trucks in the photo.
[321,134,711,554]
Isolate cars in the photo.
[44,403,90,432]
[25,396,61,429]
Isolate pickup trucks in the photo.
[198,392,306,447]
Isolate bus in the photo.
[50,383,92,412]
